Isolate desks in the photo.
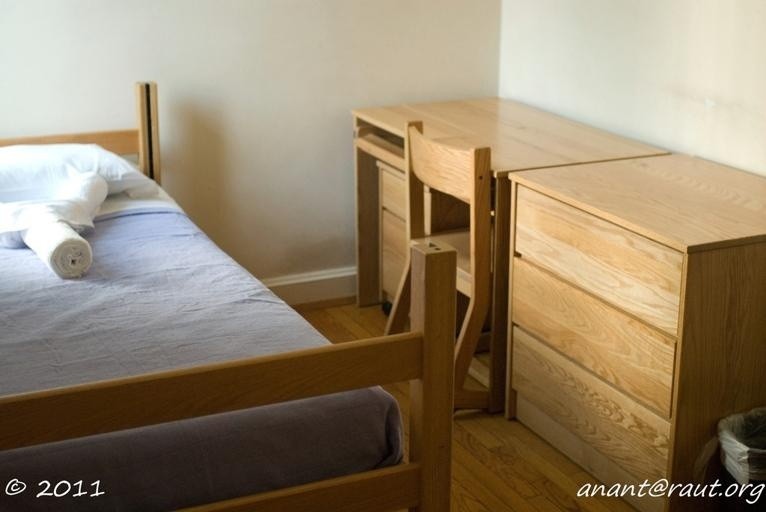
[349,101,672,413]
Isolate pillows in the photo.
[0,141,159,198]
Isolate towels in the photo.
[21,209,92,278]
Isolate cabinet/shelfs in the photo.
[375,160,407,305]
[501,155,766,512]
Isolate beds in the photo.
[1,81,458,511]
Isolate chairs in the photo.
[379,117,496,419]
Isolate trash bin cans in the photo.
[717,406,765,512]
